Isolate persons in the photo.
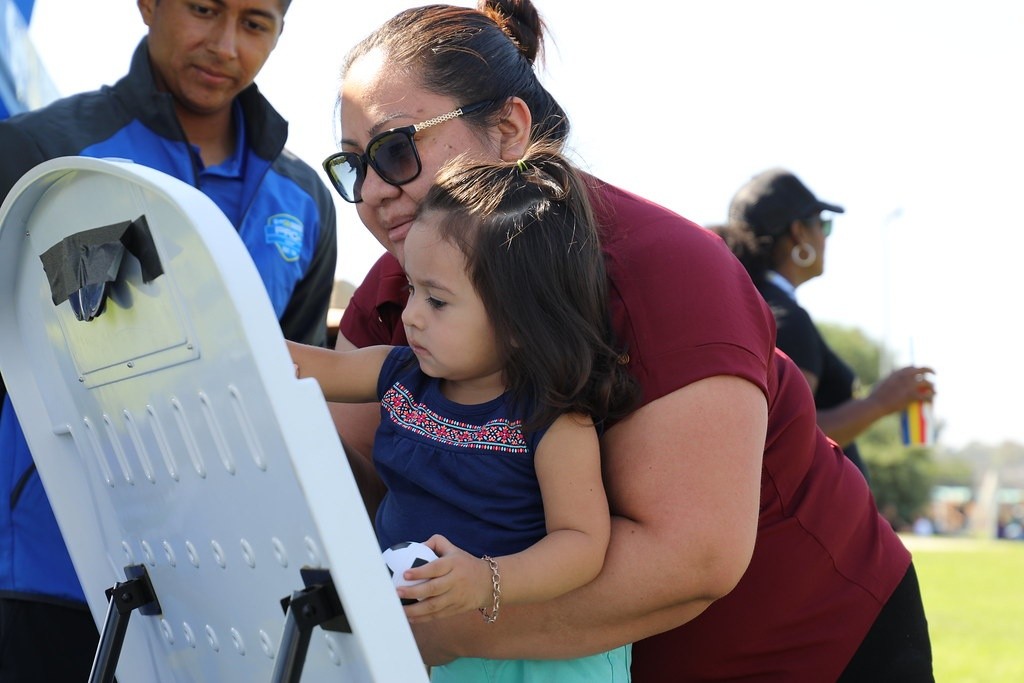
[284,137,633,683]
[703,168,937,486]
[0,0,337,683]
[322,0,935,683]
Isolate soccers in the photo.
[382,541,439,606]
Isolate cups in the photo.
[896,381,931,447]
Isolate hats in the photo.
[729,169,844,245]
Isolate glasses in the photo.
[804,219,832,236]
[321,98,495,204]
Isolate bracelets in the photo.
[478,555,501,625]
